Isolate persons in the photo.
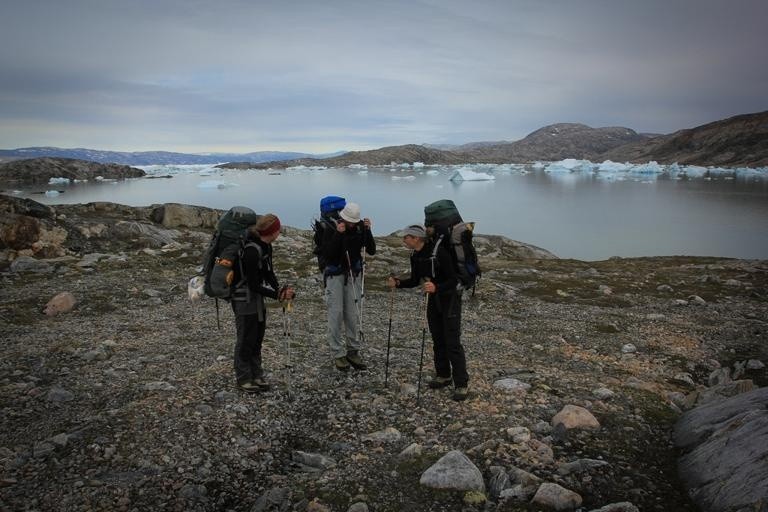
[314,203,376,370]
[229,214,296,393]
[386,226,470,400]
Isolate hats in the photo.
[397,225,427,237]
[340,202,361,224]
[255,214,280,236]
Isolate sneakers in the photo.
[253,377,269,390]
[335,357,350,372]
[347,351,367,370]
[429,376,452,389]
[238,381,259,393]
[454,384,469,401]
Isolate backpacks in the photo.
[312,196,361,274]
[203,206,264,298]
[410,199,481,289]
